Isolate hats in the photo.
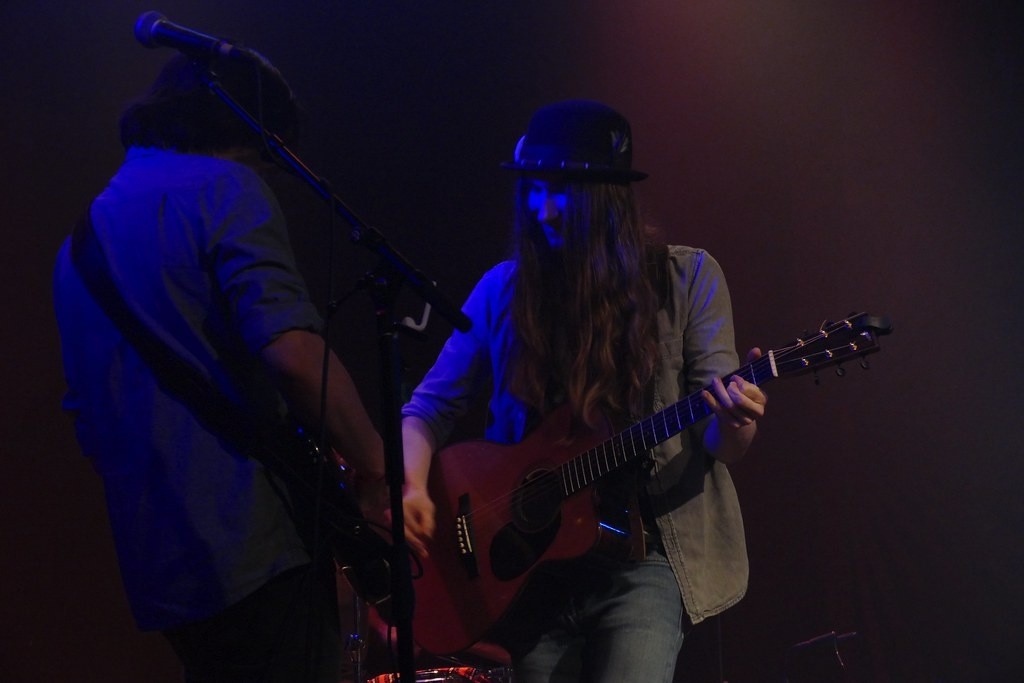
[500,101,649,185]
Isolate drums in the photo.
[365,665,496,683]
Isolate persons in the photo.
[57,47,390,683]
[403,98,769,683]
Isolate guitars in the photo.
[240,343,412,628]
[384,310,894,659]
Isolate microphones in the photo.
[131,9,245,66]
